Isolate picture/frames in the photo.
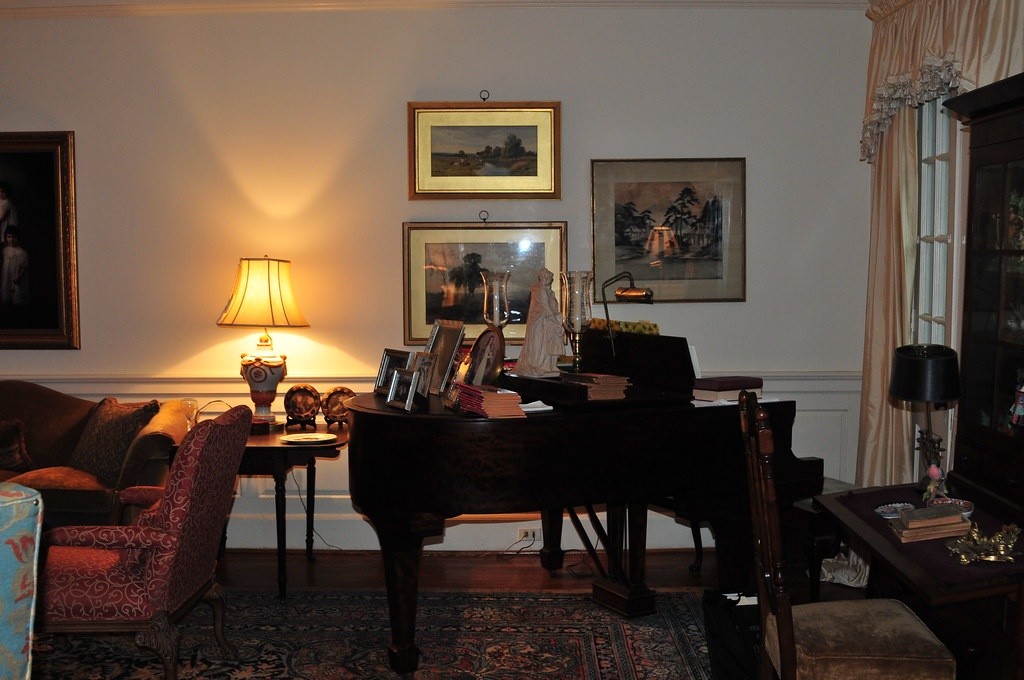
[443,355,475,410]
[413,352,438,398]
[0,131,81,350]
[374,347,415,397]
[423,318,466,396]
[405,102,562,200]
[384,367,421,412]
[468,325,506,385]
[590,157,748,304]
[402,222,569,346]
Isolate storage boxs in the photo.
[693,374,763,402]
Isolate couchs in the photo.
[0,380,198,531]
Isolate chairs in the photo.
[735,388,956,680]
[35,405,252,680]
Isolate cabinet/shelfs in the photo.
[942,71,1024,528]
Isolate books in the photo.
[560,372,633,400]
[457,381,527,419]
[887,504,971,544]
[693,376,763,402]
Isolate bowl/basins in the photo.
[927,498,974,518]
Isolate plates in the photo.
[874,503,914,518]
[279,433,337,442]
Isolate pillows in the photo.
[0,419,34,472]
[70,397,160,487]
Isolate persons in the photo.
[510,268,565,375]
[472,335,495,386]
[1,226,28,304]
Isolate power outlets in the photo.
[518,528,540,541]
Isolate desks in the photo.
[167,422,350,604]
[812,477,1024,664]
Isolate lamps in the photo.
[888,343,962,494]
[601,272,654,369]
[217,255,312,436]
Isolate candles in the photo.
[575,272,582,333]
[493,281,500,326]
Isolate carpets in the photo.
[30,591,713,680]
